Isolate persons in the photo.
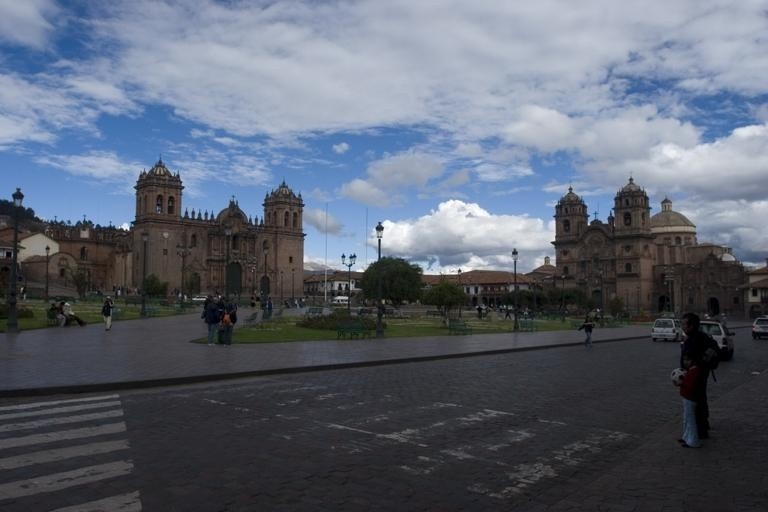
[100,296,114,333]
[201,295,237,347]
[670,312,722,448]
[49,300,87,328]
[578,314,595,349]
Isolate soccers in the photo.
[670,367,688,386]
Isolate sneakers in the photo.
[207,342,231,347]
[678,439,690,448]
[105,324,112,331]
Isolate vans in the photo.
[752,316,768,340]
[680,321,736,361]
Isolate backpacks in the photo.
[703,335,721,369]
[223,314,231,325]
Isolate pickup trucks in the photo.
[305,307,332,316]
[420,285,433,290]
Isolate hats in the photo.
[106,296,110,298]
[213,297,219,304]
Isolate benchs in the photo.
[305,308,393,317]
[46,309,76,325]
[518,317,538,332]
[337,326,371,338]
[242,306,285,328]
[426,310,443,320]
[449,317,473,335]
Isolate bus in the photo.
[332,296,351,305]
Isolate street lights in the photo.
[559,274,567,320]
[457,268,462,284]
[175,244,194,306]
[511,247,519,329]
[375,221,384,260]
[263,247,269,312]
[45,245,52,303]
[340,251,357,308]
[6,186,24,333]
[140,231,149,316]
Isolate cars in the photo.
[192,295,208,301]
[651,318,681,342]
